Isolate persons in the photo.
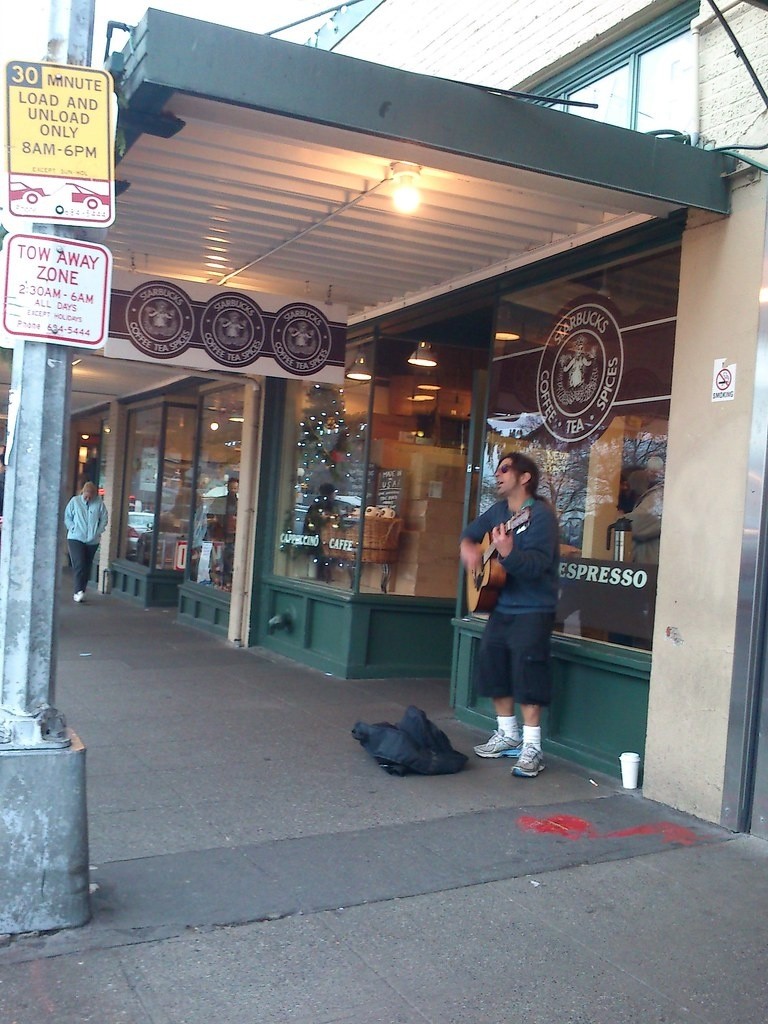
[65,480,109,603]
[458,452,560,780]
[207,478,239,591]
[302,482,337,583]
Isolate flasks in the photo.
[606,518,633,562]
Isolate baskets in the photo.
[319,514,404,564]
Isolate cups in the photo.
[352,507,396,518]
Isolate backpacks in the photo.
[351,706,468,776]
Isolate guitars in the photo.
[465,505,532,613]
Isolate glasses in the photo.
[495,464,513,475]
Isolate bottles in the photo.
[619,751,640,789]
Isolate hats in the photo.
[319,483,337,498]
[80,482,96,495]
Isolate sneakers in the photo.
[73,590,85,603]
[474,729,523,758]
[510,743,546,778]
